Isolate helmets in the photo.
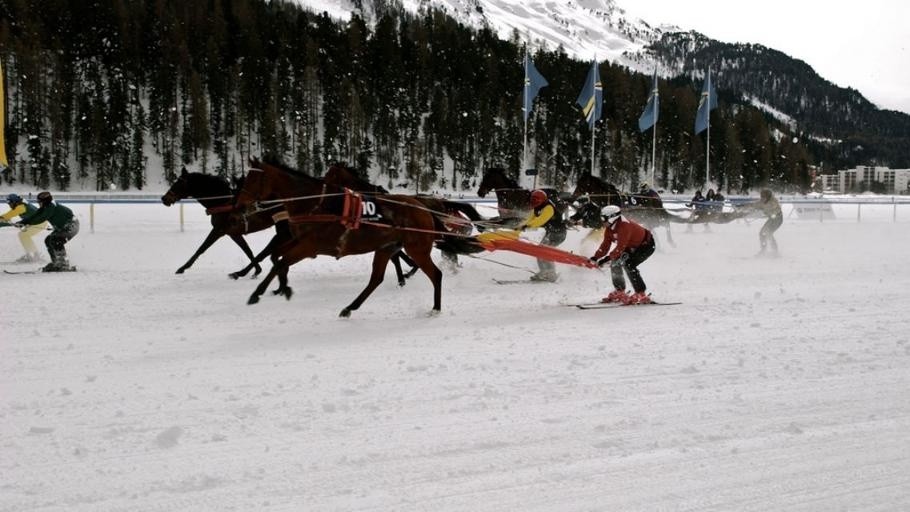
[6,194,22,205]
[600,204,621,224]
[530,190,547,208]
[35,192,52,205]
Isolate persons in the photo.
[640,184,662,208]
[0,194,46,262]
[513,190,567,282]
[744,191,782,255]
[15,192,80,272]
[591,205,655,303]
[686,190,724,216]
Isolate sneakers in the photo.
[607,291,650,303]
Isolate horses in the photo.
[571,171,673,246]
[217,155,445,318]
[160,163,274,281]
[478,167,570,231]
[322,156,484,281]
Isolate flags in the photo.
[523,57,549,124]
[638,70,660,132]
[695,71,719,135]
[577,61,603,130]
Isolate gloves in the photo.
[590,256,610,265]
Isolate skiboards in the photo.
[558,290,683,309]
[492,277,567,284]
[4,266,77,274]
[0,258,50,266]
